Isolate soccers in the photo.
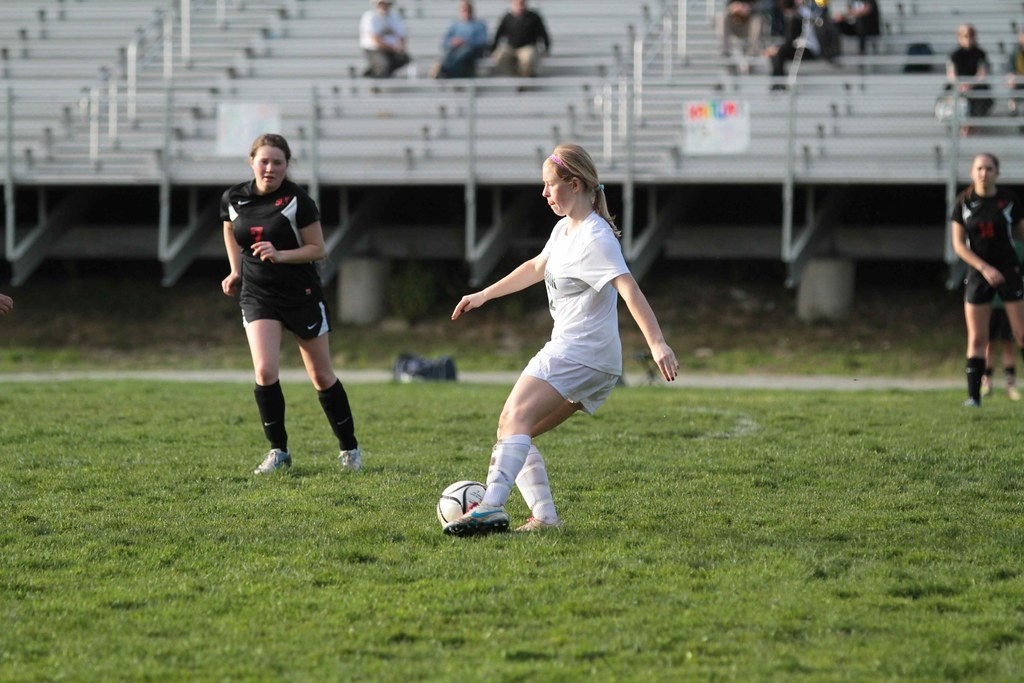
[436,480,488,529]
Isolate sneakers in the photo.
[253,447,293,476]
[341,448,362,472]
[979,387,993,399]
[443,504,510,534]
[512,516,560,533]
[962,397,981,409]
[1007,387,1020,401]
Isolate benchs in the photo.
[1,0,1022,185]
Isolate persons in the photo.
[766,1,825,90]
[432,0,488,77]
[219,133,364,481]
[489,0,548,91]
[949,152,1024,408]
[1004,29,1024,114]
[443,143,680,535]
[945,21,996,137]
[715,0,764,57]
[357,0,410,92]
[977,239,1024,402]
[830,0,880,55]
